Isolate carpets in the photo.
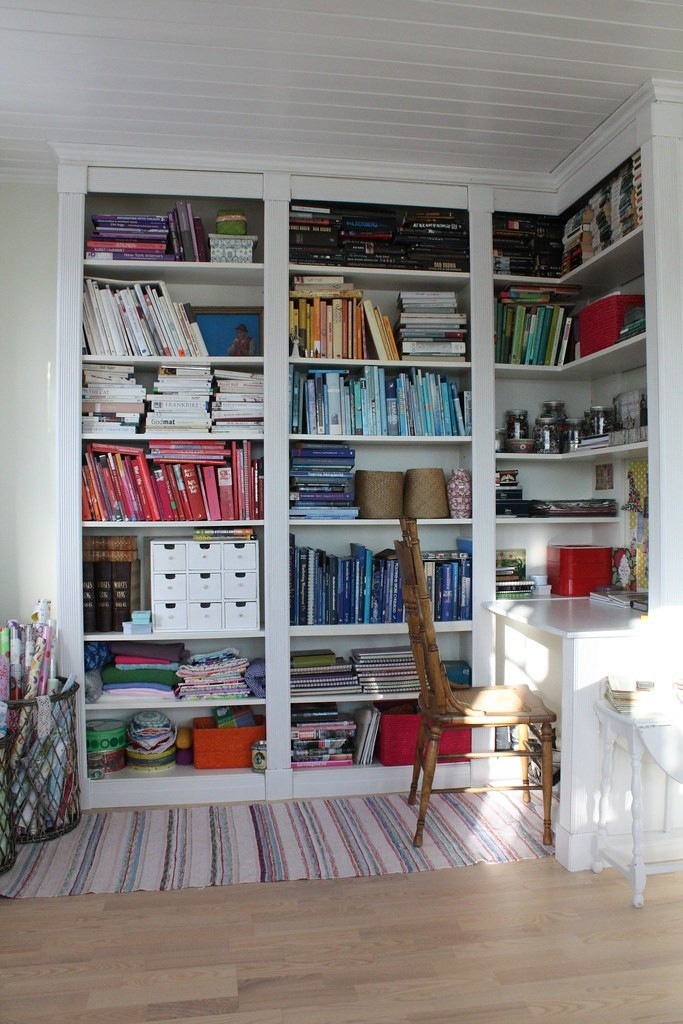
[0,789,558,913]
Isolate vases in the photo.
[447,468,472,519]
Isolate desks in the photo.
[589,698,683,909]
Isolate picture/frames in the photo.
[193,306,264,357]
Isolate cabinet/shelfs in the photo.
[44,78,683,874]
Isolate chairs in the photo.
[394,517,557,849]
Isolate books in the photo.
[575,434,609,451]
[82,275,208,357]
[493,150,643,278]
[289,538,472,626]
[495,284,579,366]
[290,645,432,696]
[289,444,360,520]
[288,365,472,435]
[589,591,648,612]
[496,550,533,599]
[289,278,467,363]
[289,206,470,272]
[615,317,646,344]
[83,536,141,632]
[86,200,207,262]
[82,440,264,523]
[82,365,264,434]
[291,703,382,769]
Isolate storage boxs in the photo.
[132,610,150,624]
[578,294,645,358]
[546,544,613,597]
[608,425,648,446]
[122,621,153,634]
[371,700,472,765]
[191,714,266,769]
[208,238,254,263]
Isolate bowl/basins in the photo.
[530,575,548,586]
[507,439,534,452]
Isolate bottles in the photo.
[495,427,506,453]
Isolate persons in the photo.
[227,325,255,357]
[291,335,300,358]
[289,333,293,356]
